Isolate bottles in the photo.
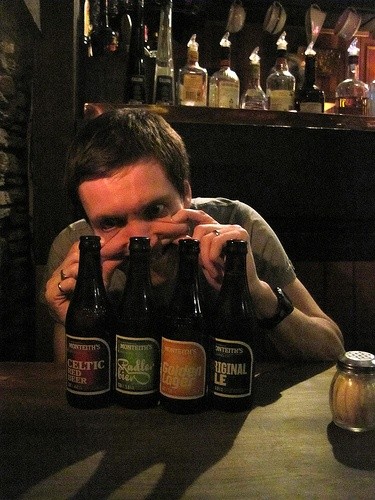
[240,45,269,110]
[159,238,210,414]
[335,39,370,116]
[296,40,325,114]
[328,350,375,432]
[176,33,207,107]
[91,0,152,58]
[208,31,239,108]
[207,239,258,412]
[112,236,161,410]
[64,235,115,410]
[123,0,175,107]
[265,31,295,110]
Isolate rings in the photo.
[213,229,222,238]
[57,282,64,293]
[60,269,69,281]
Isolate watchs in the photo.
[256,287,294,330]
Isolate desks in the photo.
[0,362,374,500]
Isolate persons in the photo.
[39,108,346,362]
